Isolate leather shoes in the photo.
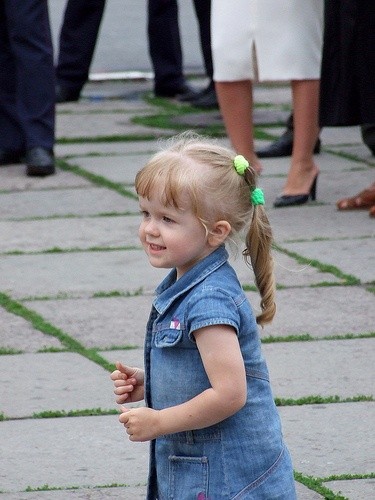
[250,128,320,158]
[153,83,198,100]
[194,80,221,109]
[53,83,80,102]
[24,145,55,175]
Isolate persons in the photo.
[255,0,375,158]
[0,0,58,177]
[172,0,222,108]
[336,182,375,218]
[108,130,297,500]
[51,0,201,105]
[211,0,324,209]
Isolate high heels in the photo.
[273,168,318,207]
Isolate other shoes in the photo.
[338,183,375,215]
